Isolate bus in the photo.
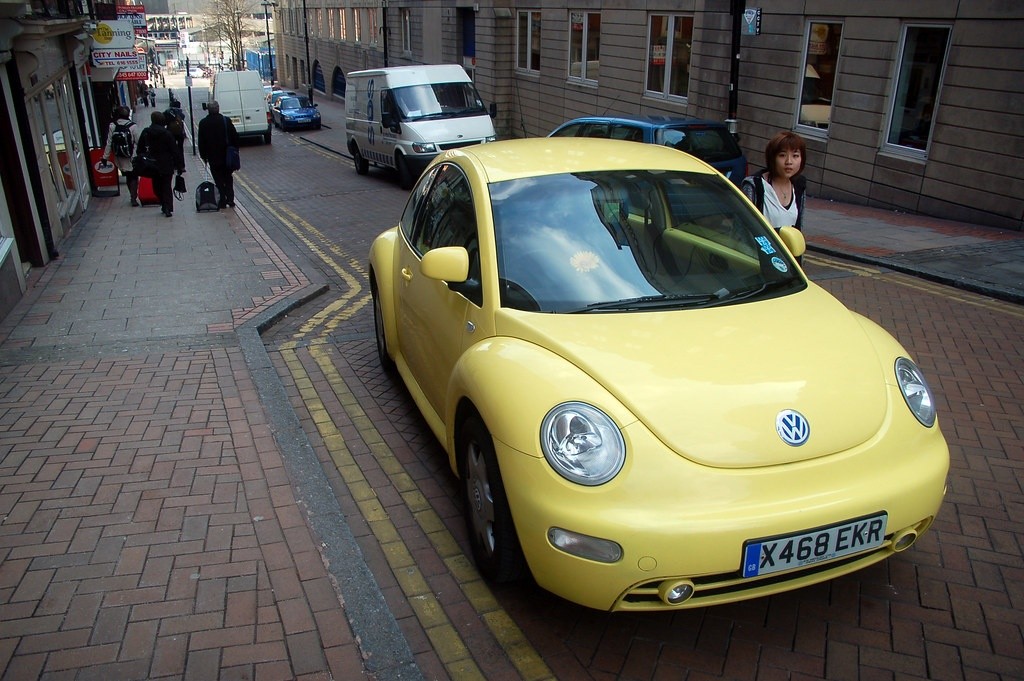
[546,115,749,190]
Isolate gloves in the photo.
[101,159,107,167]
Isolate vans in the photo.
[202,70,272,145]
[345,64,497,192]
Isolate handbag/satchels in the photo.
[173,173,187,201]
[227,146,240,169]
[132,154,151,174]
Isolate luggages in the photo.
[195,160,220,211]
[138,176,160,206]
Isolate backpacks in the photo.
[169,109,182,134]
[112,121,136,158]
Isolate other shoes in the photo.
[162,208,166,213]
[227,201,235,206]
[166,212,172,217]
[132,199,139,207]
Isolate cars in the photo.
[272,95,321,132]
[186,68,206,77]
[369,136,952,611]
[263,86,298,122]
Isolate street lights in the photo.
[235,9,245,71]
[261,1,274,87]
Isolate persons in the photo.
[161,101,186,173]
[197,100,241,208]
[136,111,179,217]
[101,106,141,207]
[737,132,807,278]
[139,79,148,107]
[147,84,156,107]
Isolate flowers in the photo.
[570,251,602,274]
